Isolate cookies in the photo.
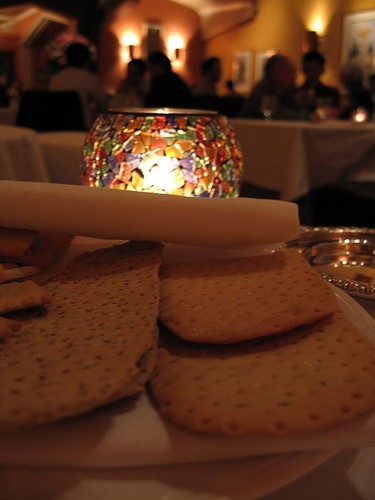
[0,227,375,435]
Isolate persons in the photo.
[116,50,242,109]
[247,51,375,122]
[50,41,112,127]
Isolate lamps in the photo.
[80,108,244,249]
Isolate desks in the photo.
[228,118,375,202]
[0,125,88,185]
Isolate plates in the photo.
[0,281,375,500]
